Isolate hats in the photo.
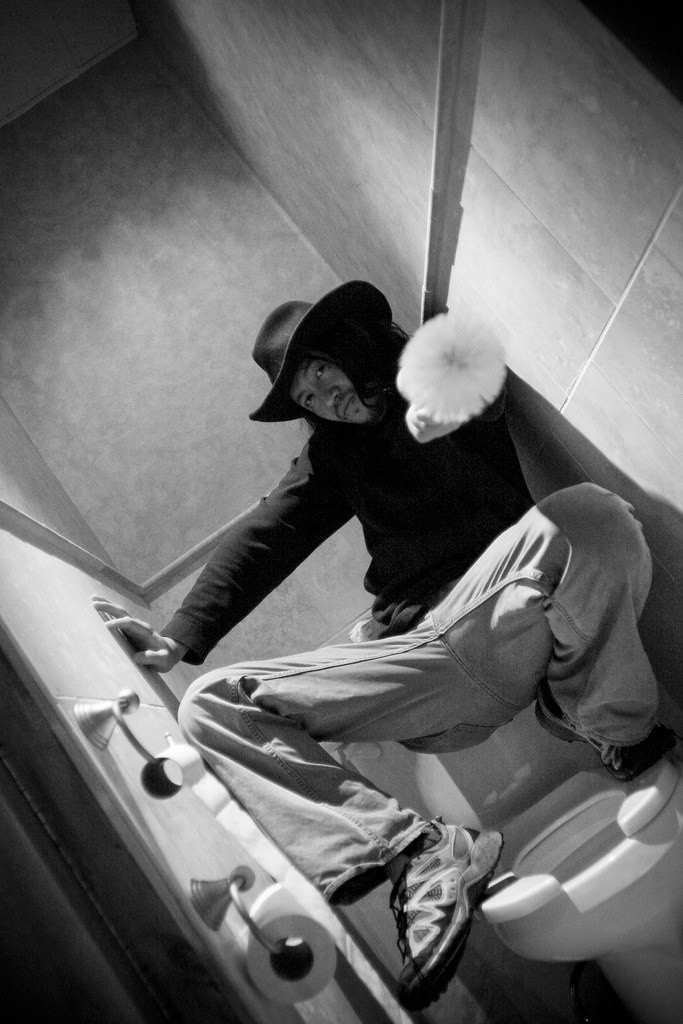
[249,280,393,424]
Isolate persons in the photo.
[91,277,680,1010]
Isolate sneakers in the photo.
[535,687,677,783]
[390,817,506,1010]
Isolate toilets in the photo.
[470,752,683,1024]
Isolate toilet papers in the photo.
[241,882,349,1006]
